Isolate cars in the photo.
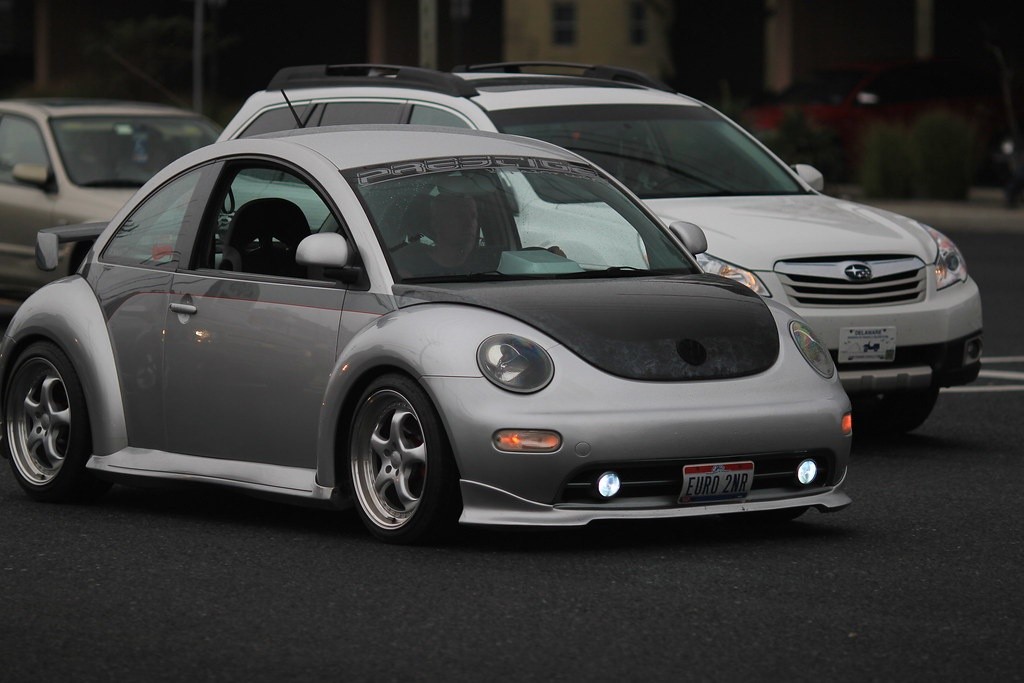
[2,123,858,541]
[0,93,225,294]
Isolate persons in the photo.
[391,175,567,285]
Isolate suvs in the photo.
[203,60,989,443]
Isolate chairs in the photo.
[217,198,312,280]
